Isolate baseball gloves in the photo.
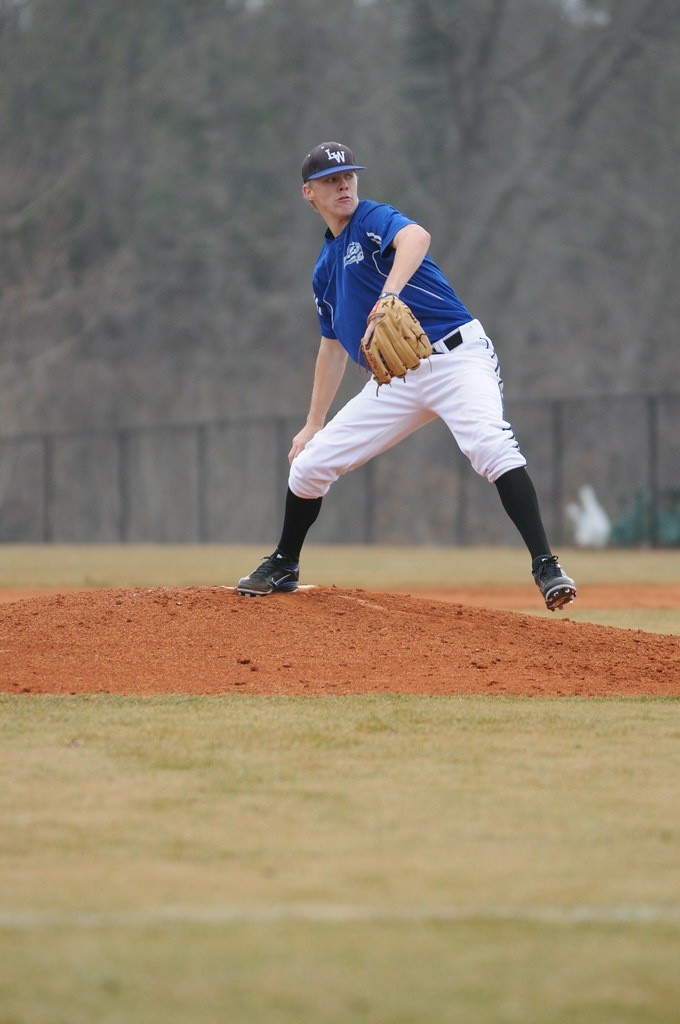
[361,299,434,393]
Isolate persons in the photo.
[237,140,576,612]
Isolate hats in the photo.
[302,140,367,184]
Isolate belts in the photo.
[431,330,463,353]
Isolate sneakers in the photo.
[531,554,577,612]
[236,547,300,596]
